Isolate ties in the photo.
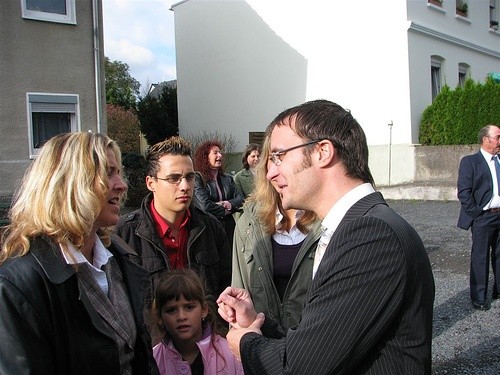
[491,156,500,198]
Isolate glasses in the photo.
[270,137,337,166]
[149,173,195,184]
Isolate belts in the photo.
[486,208,500,212]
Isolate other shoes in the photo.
[472,300,489,310]
[492,294,500,298]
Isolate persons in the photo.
[216,100,435,375]
[112,137,324,375]
[457,125,500,309]
[0,131,156,375]
